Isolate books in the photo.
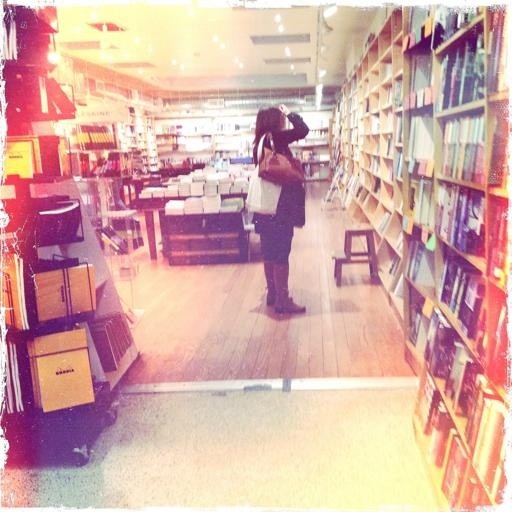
[139,162,255,216]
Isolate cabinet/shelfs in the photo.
[2,1,142,468]
[326,5,413,327]
[403,4,509,512]
[142,94,329,182]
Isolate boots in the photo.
[264,262,306,313]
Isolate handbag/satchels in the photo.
[244,131,305,215]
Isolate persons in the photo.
[252,104,309,313]
